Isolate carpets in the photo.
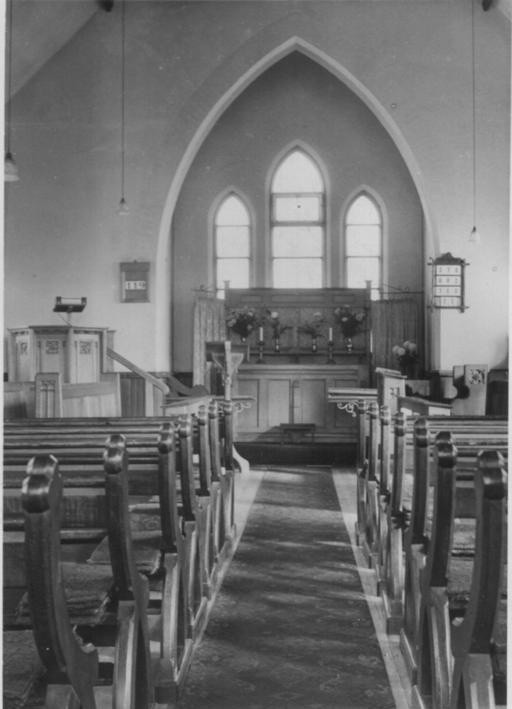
[167,462,394,709]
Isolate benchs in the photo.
[356,397,509,708]
[4,397,238,709]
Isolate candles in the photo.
[329,327,333,342]
[259,326,263,341]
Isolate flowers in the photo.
[225,305,261,341]
[334,304,369,338]
